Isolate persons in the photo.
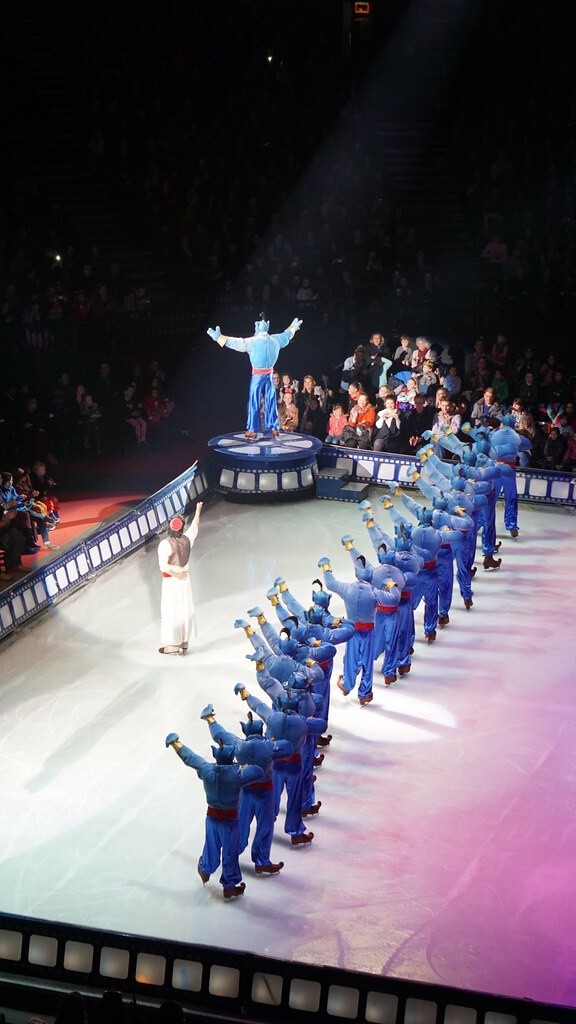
[164,411,532,903]
[0,140,176,577]
[449,0,575,315]
[92,0,436,326]
[159,502,204,655]
[285,335,576,469]
[205,312,304,439]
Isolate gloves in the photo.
[318,557,332,572]
[266,587,280,606]
[451,461,504,516]
[382,578,398,589]
[341,534,354,550]
[200,704,216,724]
[246,649,265,671]
[390,420,472,495]
[166,733,183,751]
[234,683,251,700]
[207,326,227,347]
[440,525,452,532]
[286,317,303,336]
[380,494,392,509]
[331,618,343,628]
[359,500,374,514]
[307,637,323,647]
[274,576,288,592]
[362,512,375,528]
[248,606,267,625]
[234,619,255,636]
[300,658,317,667]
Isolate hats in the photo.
[170,518,184,532]
[18,465,30,478]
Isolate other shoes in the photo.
[224,882,246,899]
[245,431,257,437]
[198,856,210,883]
[14,565,31,572]
[438,616,450,625]
[317,735,332,748]
[337,675,349,696]
[360,691,373,705]
[272,430,280,438]
[24,512,60,554]
[467,528,519,578]
[292,831,314,846]
[314,753,324,767]
[425,629,436,642]
[158,647,180,655]
[255,862,284,874]
[398,665,411,675]
[464,599,473,607]
[183,646,187,652]
[301,801,321,818]
[385,674,397,684]
